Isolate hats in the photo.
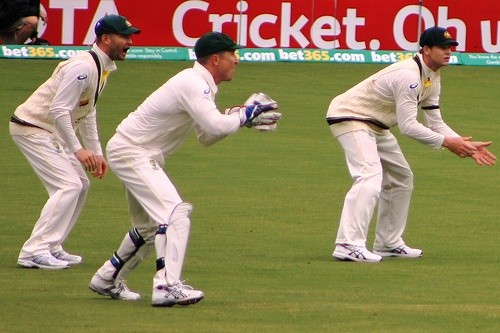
[420,27,459,47]
[94,14,141,35]
[194,31,248,57]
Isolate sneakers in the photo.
[16,253,70,269]
[373,242,423,258]
[152,282,204,306]
[89,273,141,300]
[332,244,382,262]
[51,250,82,265]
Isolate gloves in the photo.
[224,106,282,132]
[238,93,278,127]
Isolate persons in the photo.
[0,0,48,45]
[326,27,496,262]
[8,15,140,270]
[89,32,282,306]
